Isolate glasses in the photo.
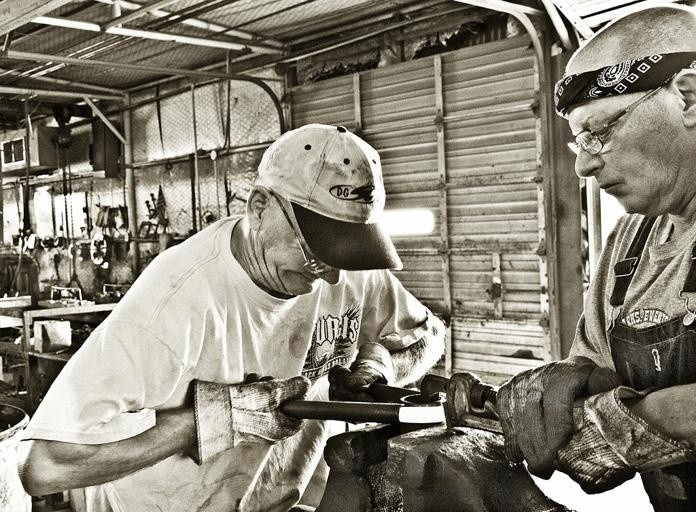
[265,188,336,276]
[566,64,679,158]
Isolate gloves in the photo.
[327,340,399,401]
[183,371,307,466]
[495,356,694,493]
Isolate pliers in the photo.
[421,374,504,435]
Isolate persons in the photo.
[18,123,447,511]
[495,2,695,512]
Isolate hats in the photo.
[253,121,405,271]
